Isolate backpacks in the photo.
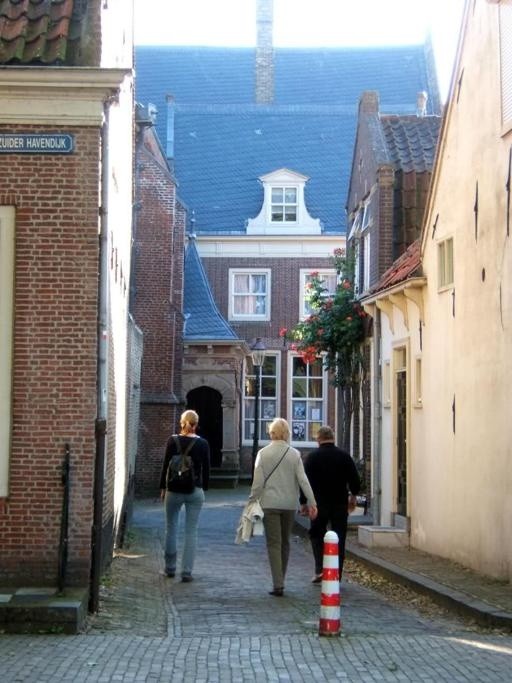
[165,433,200,495]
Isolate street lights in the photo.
[249,334,268,478]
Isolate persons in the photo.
[298,425,361,583]
[159,409,211,582]
[248,417,319,596]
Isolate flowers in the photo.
[277,248,367,389]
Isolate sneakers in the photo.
[269,587,284,597]
[182,575,195,583]
[164,567,176,577]
[312,573,324,584]
